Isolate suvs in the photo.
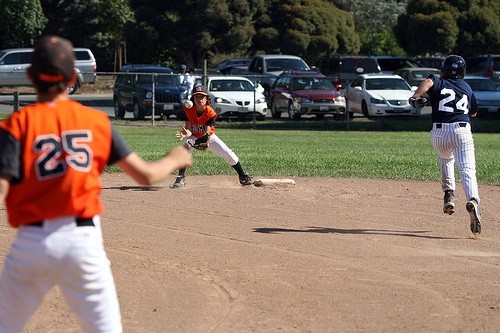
[113,65,189,121]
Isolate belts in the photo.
[436,123,467,128]
[27,215,95,228]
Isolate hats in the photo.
[189,85,210,96]
[30,36,77,84]
[179,64,186,70]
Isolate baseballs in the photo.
[185,101,193,109]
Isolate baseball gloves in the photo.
[188,133,211,150]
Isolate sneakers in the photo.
[443,192,455,215]
[466,200,482,236]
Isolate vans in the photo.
[320,56,382,98]
[0,48,98,95]
[249,54,316,110]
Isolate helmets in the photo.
[440,55,467,79]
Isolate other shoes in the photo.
[239,173,254,185]
[173,175,186,188]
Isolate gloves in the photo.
[409,97,428,109]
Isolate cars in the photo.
[270,68,346,121]
[341,73,422,119]
[198,76,268,121]
[375,55,500,90]
[460,75,500,119]
[178,58,252,103]
[395,67,442,106]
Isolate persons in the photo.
[173,86,252,188]
[409,55,482,235]
[0,36,194,333]
